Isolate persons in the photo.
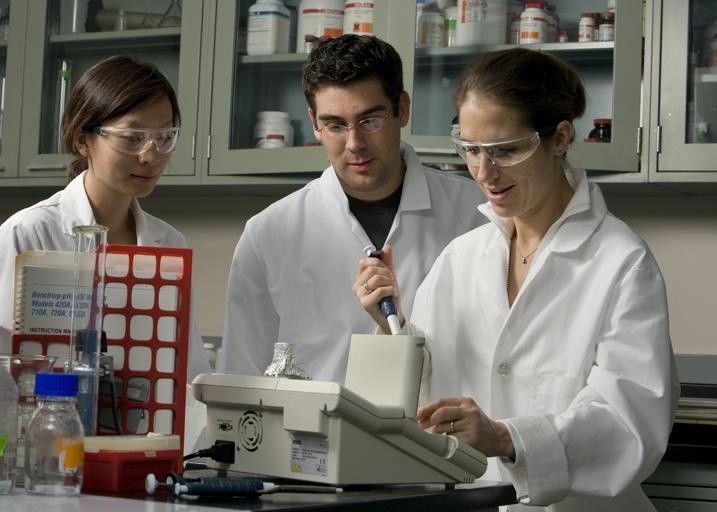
[0,55,212,382]
[351,47,676,511]
[217,34,491,382]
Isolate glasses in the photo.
[448,116,557,169]
[310,103,398,137]
[84,122,179,158]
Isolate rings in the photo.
[449,421,455,433]
[363,280,373,294]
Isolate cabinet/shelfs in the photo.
[0,0,210,196]
[649,0,717,197]
[201,0,653,197]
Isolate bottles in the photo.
[0,221,110,499]
[113,7,127,32]
[54,49,77,153]
[245,0,374,56]
[58,0,89,34]
[448,113,460,137]
[302,114,323,145]
[587,118,612,144]
[702,15,716,67]
[251,110,296,148]
[416,0,616,47]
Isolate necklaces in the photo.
[510,237,537,264]
